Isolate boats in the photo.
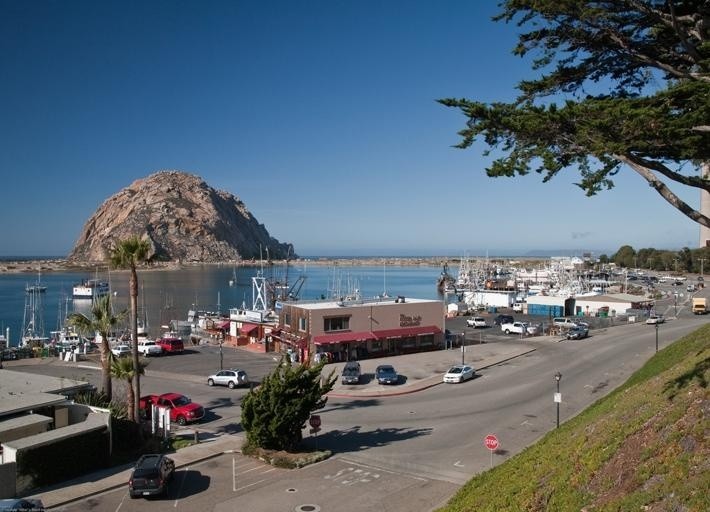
[18,264,80,346]
[137,280,147,336]
[73,279,112,296]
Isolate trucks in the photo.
[692,298,707,314]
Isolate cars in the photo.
[687,285,695,291]
[646,315,665,324]
[513,303,524,314]
[1,498,43,512]
[138,338,184,356]
[628,276,666,285]
[111,345,132,357]
[467,316,487,328]
[341,362,361,385]
[376,365,398,384]
[493,314,537,336]
[671,282,683,286]
[553,317,588,339]
[129,454,175,499]
[443,365,476,384]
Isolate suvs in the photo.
[208,368,248,389]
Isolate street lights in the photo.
[554,372,562,428]
[656,326,658,352]
[698,259,708,278]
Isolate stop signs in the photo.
[310,416,321,426]
[484,435,499,450]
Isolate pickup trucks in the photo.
[140,393,205,425]
